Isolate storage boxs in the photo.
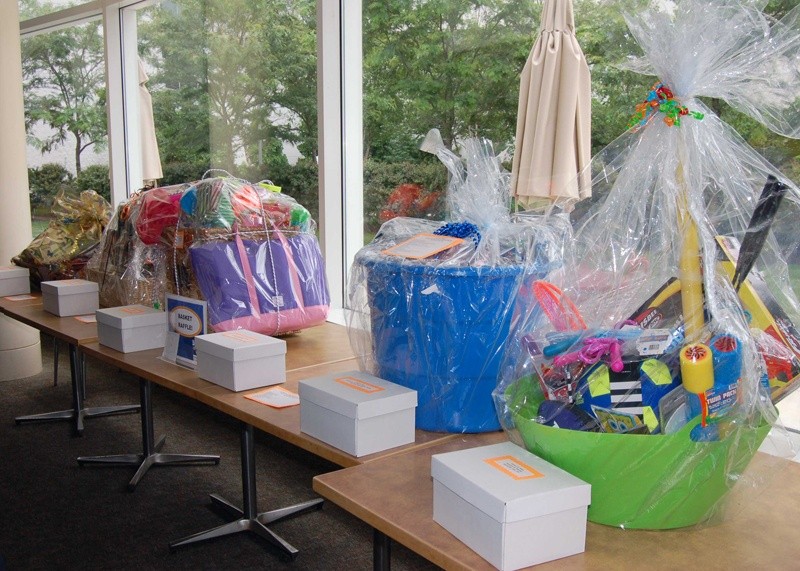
[41,279,99,317]
[194,328,288,393]
[298,370,418,458]
[95,304,167,353]
[431,441,592,571]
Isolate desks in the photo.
[0,292,800,571]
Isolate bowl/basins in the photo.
[504,369,779,530]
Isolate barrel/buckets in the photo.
[354,252,565,434]
